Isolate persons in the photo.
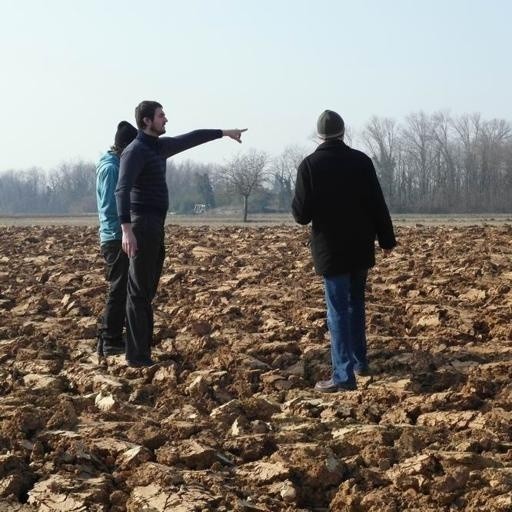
[114,101,248,366]
[291,109,398,394]
[96,121,138,355]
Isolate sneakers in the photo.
[96,345,125,356]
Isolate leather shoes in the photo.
[314,370,369,393]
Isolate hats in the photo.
[317,110,345,138]
[115,121,138,150]
